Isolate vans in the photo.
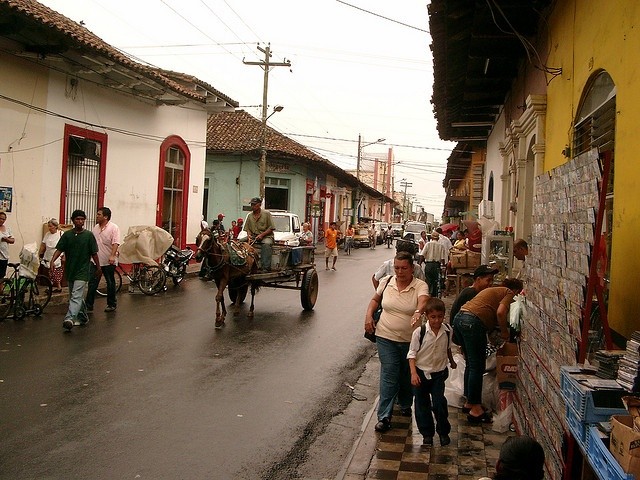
[236,209,303,247]
[402,222,427,243]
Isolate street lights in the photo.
[392,177,407,200]
[382,161,404,195]
[260,104,284,209]
[356,134,386,181]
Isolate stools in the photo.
[456,268,476,295]
[446,275,457,296]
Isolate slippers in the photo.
[466,412,493,422]
[463,404,496,414]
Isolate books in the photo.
[616,331,640,390]
[594,349,625,379]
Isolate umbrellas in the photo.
[439,223,459,235]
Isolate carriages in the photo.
[195,221,318,327]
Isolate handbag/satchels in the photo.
[363,305,383,343]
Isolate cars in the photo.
[367,225,386,245]
[390,223,403,236]
[376,223,390,237]
[352,227,373,247]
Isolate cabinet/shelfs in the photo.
[481,235,514,287]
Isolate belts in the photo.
[459,310,476,316]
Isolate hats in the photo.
[431,232,440,239]
[249,197,261,204]
[237,218,244,223]
[472,264,499,279]
[218,213,225,218]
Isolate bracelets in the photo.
[415,310,421,313]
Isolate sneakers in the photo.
[86,307,92,313]
[441,435,453,446]
[421,436,435,444]
[53,289,62,293]
[332,267,336,271]
[73,314,90,327]
[105,304,117,312]
[62,318,74,333]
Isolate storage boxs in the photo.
[609,414,640,480]
[450,250,467,268]
[496,342,518,390]
[466,249,481,269]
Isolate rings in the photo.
[413,318,415,320]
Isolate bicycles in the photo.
[345,237,353,255]
[0,263,53,322]
[94,251,166,297]
[368,234,375,250]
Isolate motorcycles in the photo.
[382,231,396,248]
[152,244,195,293]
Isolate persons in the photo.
[230,221,236,240]
[38,218,66,293]
[418,208,428,223]
[243,197,275,272]
[384,225,395,245]
[325,222,338,271]
[464,229,469,248]
[85,207,120,313]
[232,218,244,240]
[345,224,355,255]
[372,240,427,290]
[210,220,221,239]
[365,251,429,433]
[406,298,458,446]
[512,238,528,292]
[50,210,102,331]
[479,435,546,480]
[436,228,454,290]
[450,265,499,329]
[218,214,225,232]
[0,212,15,297]
[453,277,524,421]
[301,223,313,246]
[447,231,466,273]
[368,224,377,250]
[418,231,447,298]
[417,231,427,265]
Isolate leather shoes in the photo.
[401,408,412,418]
[376,421,393,433]
